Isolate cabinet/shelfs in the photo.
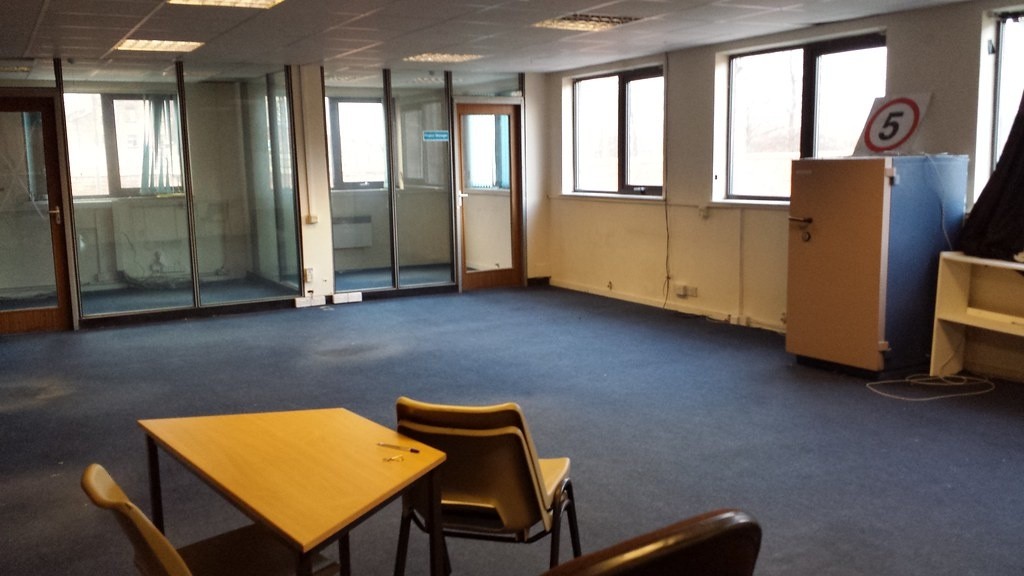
[929,251,1024,382]
[785,155,968,372]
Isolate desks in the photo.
[136,407,447,576]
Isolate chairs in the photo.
[82,462,340,576]
[393,396,582,576]
[544,510,761,576]
